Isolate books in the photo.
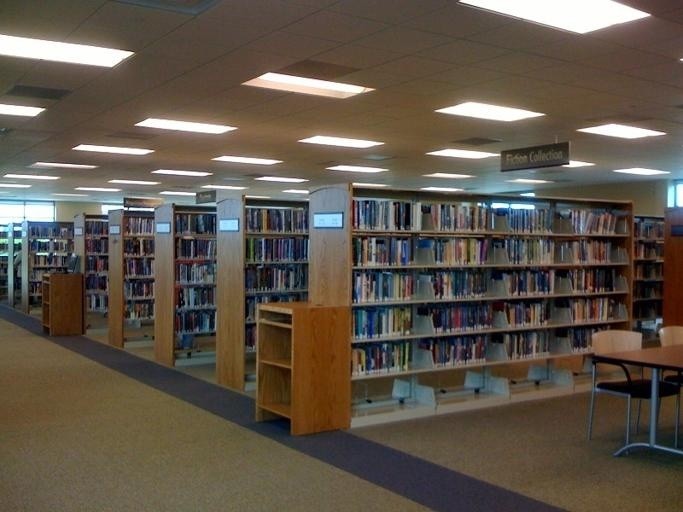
[633,218,665,323]
[14,230,22,306]
[124,215,155,320]
[86,216,108,313]
[175,211,217,352]
[29,224,74,307]
[351,200,629,376]
[0,231,8,297]
[244,207,309,354]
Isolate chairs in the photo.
[659,326,683,427]
[588,329,680,456]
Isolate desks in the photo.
[592,344,683,457]
